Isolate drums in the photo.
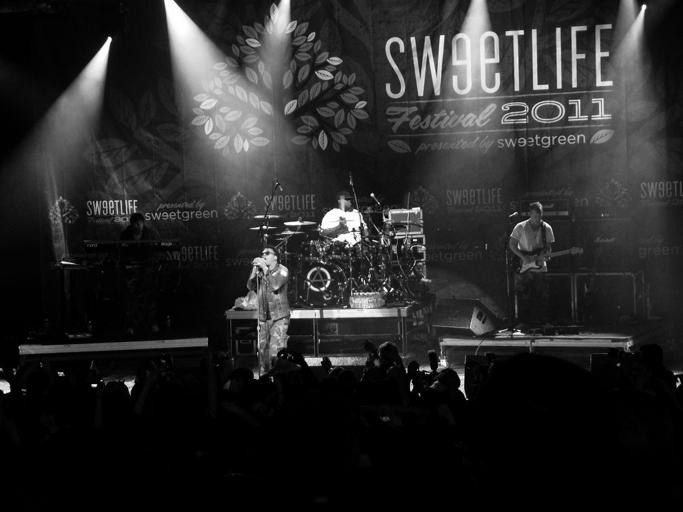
[301,240,330,261]
[298,260,346,307]
[353,240,376,262]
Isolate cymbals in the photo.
[255,214,280,219]
[249,225,277,231]
[285,219,317,227]
[275,228,304,237]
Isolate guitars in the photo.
[513,246,583,273]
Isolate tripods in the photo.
[472,220,535,340]
[288,238,312,309]
[354,249,384,293]
[335,253,361,307]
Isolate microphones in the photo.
[367,207,372,217]
[508,211,518,218]
[272,176,284,192]
[370,192,380,204]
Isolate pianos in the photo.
[81,238,172,251]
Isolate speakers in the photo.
[430,298,503,336]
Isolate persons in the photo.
[1,340,683,512]
[317,192,369,278]
[247,247,290,376]
[118,212,160,265]
[508,201,555,336]
